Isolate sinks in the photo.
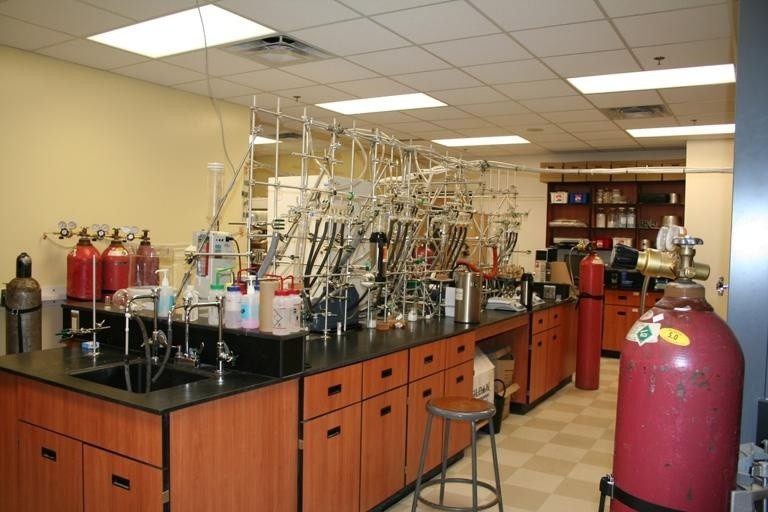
[68,357,213,395]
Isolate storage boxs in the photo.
[475,344,520,422]
[540,160,686,182]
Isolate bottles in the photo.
[521,272,533,310]
[182,267,302,336]
[595,187,636,228]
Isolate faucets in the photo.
[167,296,205,368]
[123,291,161,361]
[185,296,226,374]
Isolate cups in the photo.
[367,307,377,329]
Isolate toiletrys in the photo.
[155,267,174,318]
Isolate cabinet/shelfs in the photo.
[546,181,685,354]
[548,304,562,398]
[298,330,475,512]
[16,374,163,512]
[478,301,548,415]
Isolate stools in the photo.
[411,397,503,512]
